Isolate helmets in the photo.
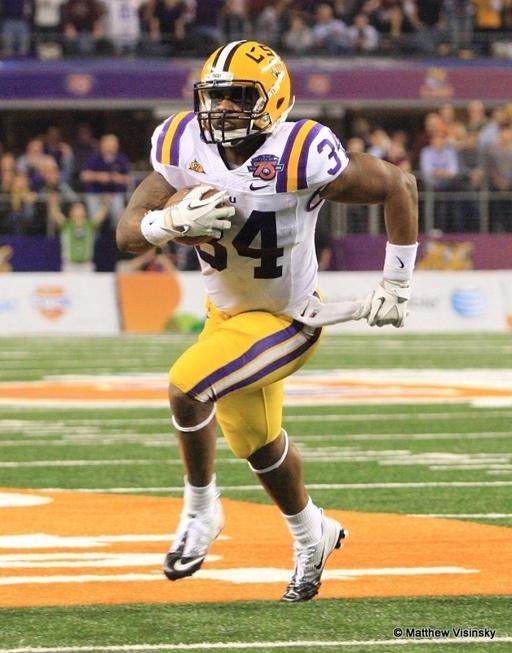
[201,39,292,131]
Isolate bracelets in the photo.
[380,239,421,281]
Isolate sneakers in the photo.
[279,508,348,600]
[162,500,226,580]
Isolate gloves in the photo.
[351,278,412,329]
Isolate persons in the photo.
[0,0,510,270]
[114,37,419,603]
